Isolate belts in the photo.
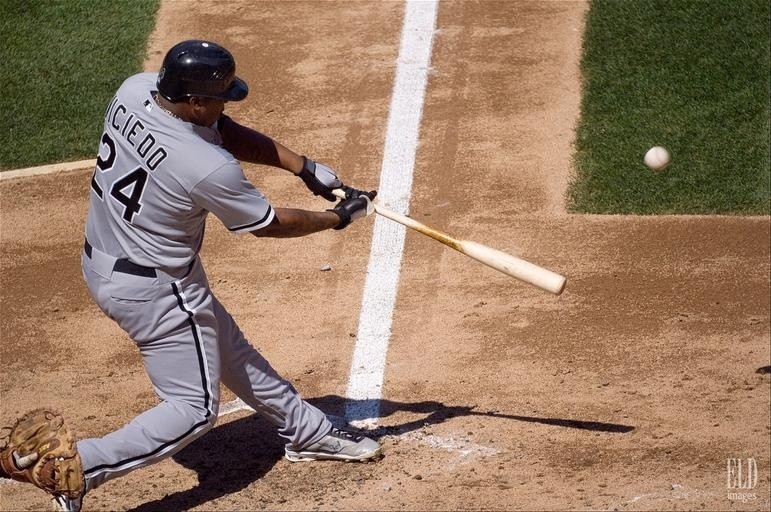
[84,239,157,278]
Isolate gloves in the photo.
[296,155,344,203]
[325,188,377,229]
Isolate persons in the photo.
[56,41,382,512]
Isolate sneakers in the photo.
[284,426,382,463]
[54,493,83,512]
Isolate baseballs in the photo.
[644,146,669,169]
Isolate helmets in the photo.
[157,40,249,102]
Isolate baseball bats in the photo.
[332,189,567,296]
